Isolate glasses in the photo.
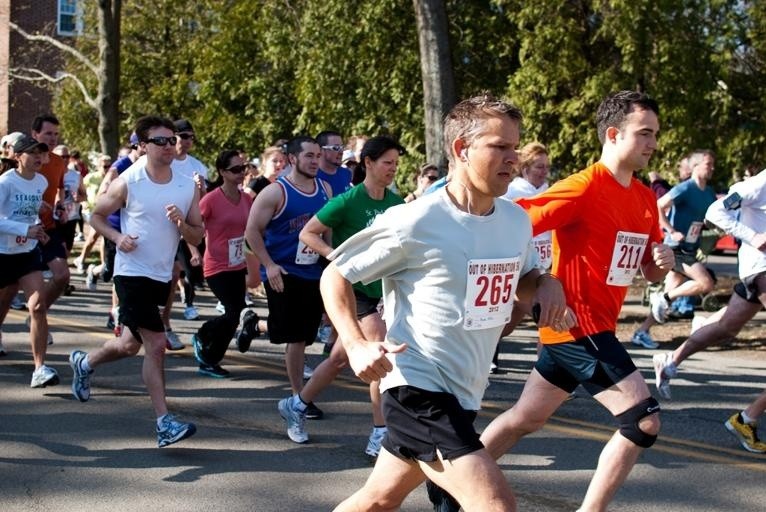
[178,131,195,141]
[62,154,70,159]
[321,144,345,152]
[145,135,178,147]
[426,175,438,181]
[224,163,247,175]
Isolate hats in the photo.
[130,130,139,148]
[14,135,49,152]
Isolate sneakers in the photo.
[24,317,53,345]
[70,349,93,401]
[165,330,232,378]
[365,431,387,459]
[302,358,314,380]
[10,295,25,310]
[630,330,660,349]
[317,320,336,345]
[725,411,766,453]
[30,364,60,387]
[652,353,688,400]
[235,308,259,353]
[183,305,200,321]
[155,410,198,448]
[649,291,671,324]
[278,393,323,444]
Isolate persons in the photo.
[182,119,440,458]
[319,91,566,511]
[69,118,204,446]
[633,148,766,453]
[492,139,574,399]
[1,113,209,388]
[426,90,676,511]
